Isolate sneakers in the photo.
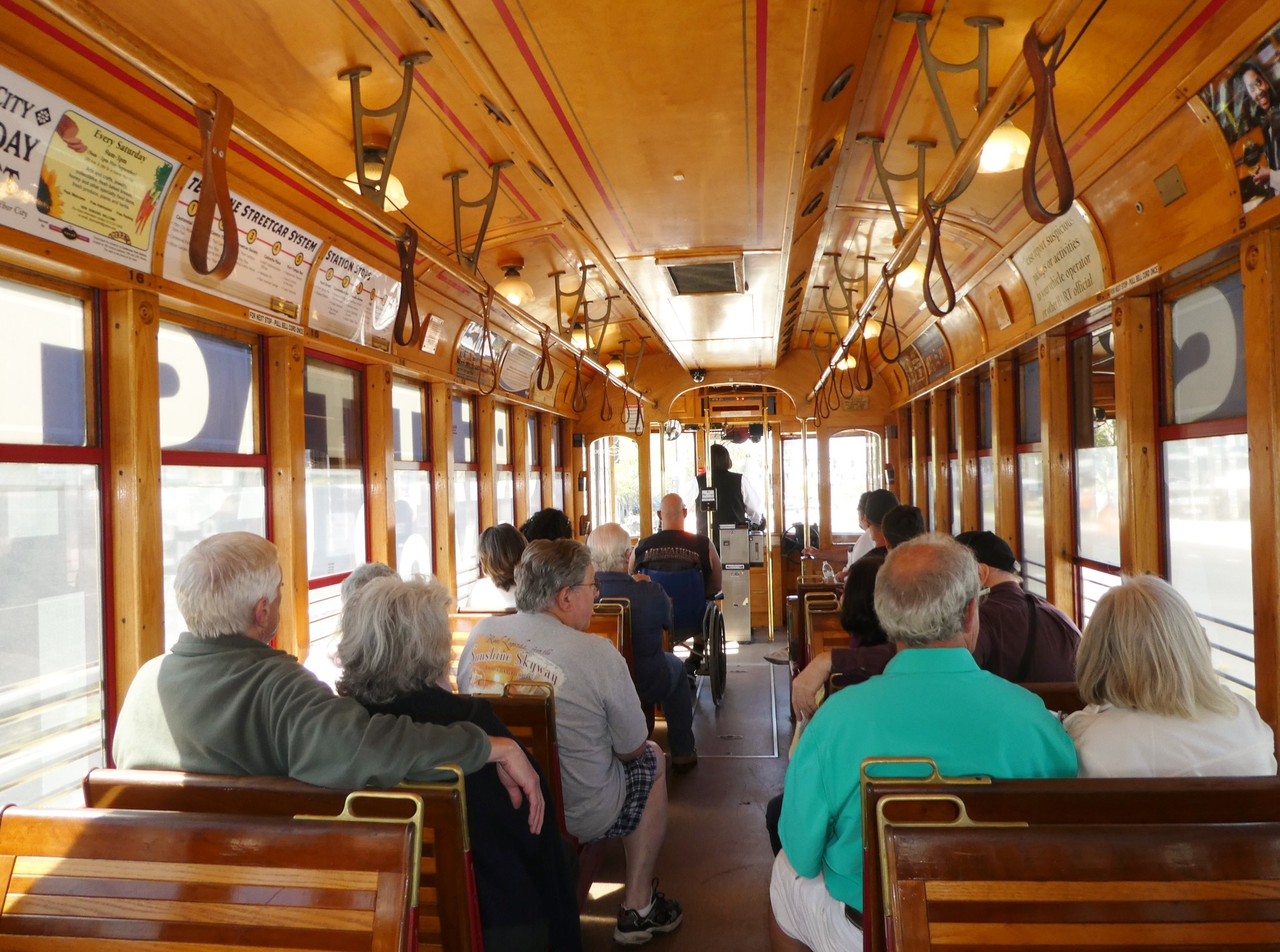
[671,749,698,771]
[615,877,684,946]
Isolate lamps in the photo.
[863,316,881,339]
[835,352,856,370]
[973,87,1032,173]
[605,351,628,377]
[567,315,596,350]
[893,228,927,289]
[337,133,410,214]
[493,258,535,307]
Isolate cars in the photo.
[653,501,660,511]
[618,501,639,515]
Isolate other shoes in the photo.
[682,659,697,689]
[764,650,789,665]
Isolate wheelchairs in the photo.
[637,565,727,706]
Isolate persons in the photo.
[766,530,1083,857]
[334,573,581,952]
[465,523,528,609]
[681,444,762,644]
[764,491,876,665]
[881,503,926,553]
[304,563,400,698]
[457,540,685,944]
[770,533,1078,952]
[628,494,722,670]
[586,523,698,771]
[112,532,546,835]
[519,508,573,545]
[1239,62,1280,196]
[839,489,900,648]
[1062,576,1277,778]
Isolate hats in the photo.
[954,530,1019,573]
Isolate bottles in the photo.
[822,561,837,583]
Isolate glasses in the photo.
[555,579,600,594]
[960,588,991,621]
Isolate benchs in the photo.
[0,575,667,952]
[797,574,1280,952]
